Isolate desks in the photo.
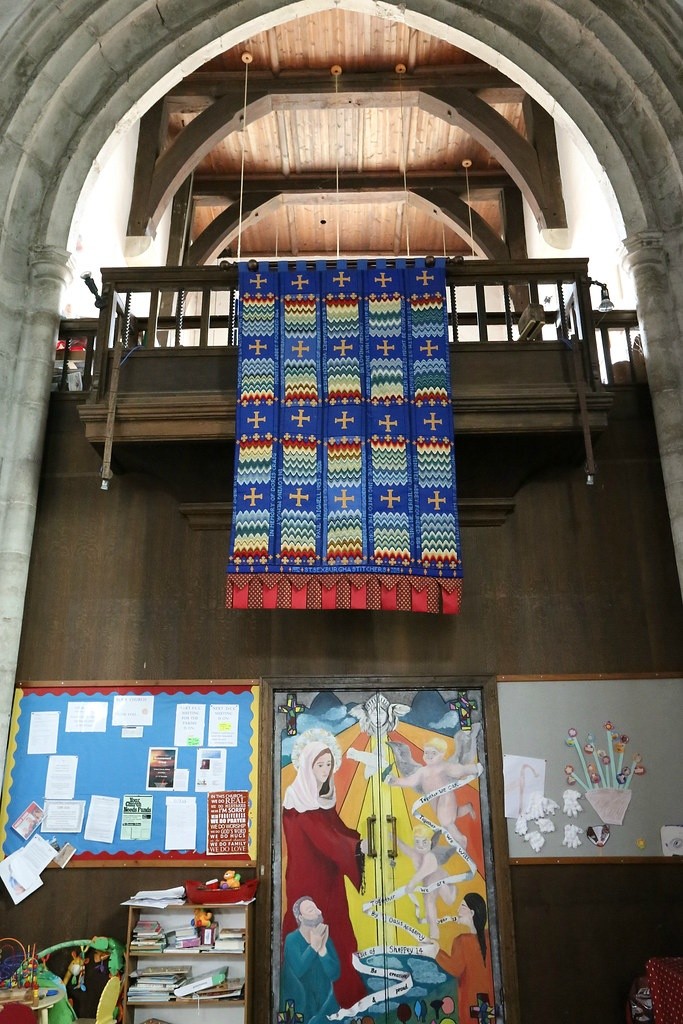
[0,987,63,1024]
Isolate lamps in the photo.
[80,271,107,307]
[589,276,614,313]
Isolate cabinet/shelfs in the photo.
[123,901,253,1024]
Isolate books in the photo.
[130,921,246,953]
[128,965,245,1002]
